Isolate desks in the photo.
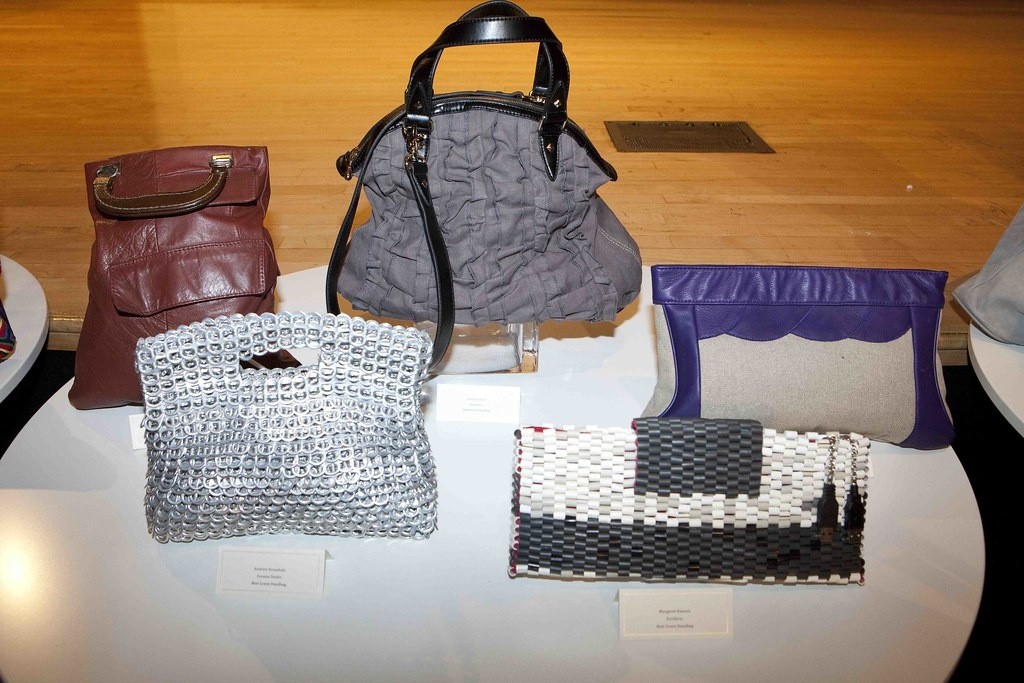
[0,253,1024,683]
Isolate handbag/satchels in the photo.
[132,310,437,542]
[69,145,307,410]
[507,418,870,588]
[643,264,953,450]
[324,0,643,370]
